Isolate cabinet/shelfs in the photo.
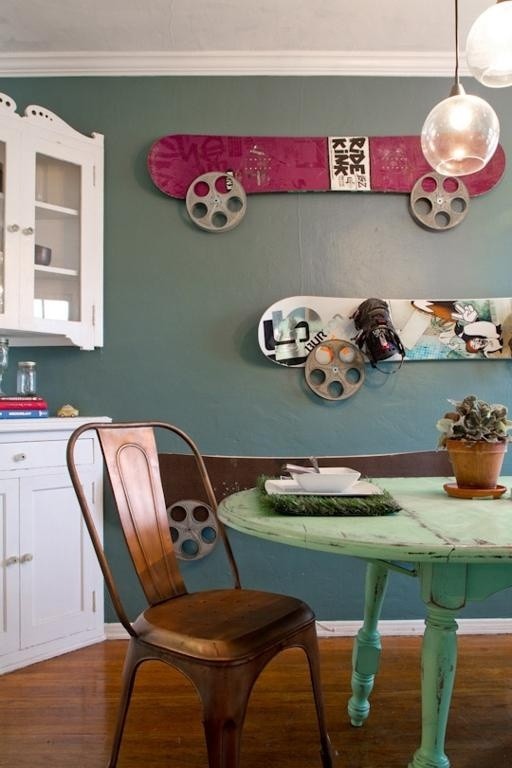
[1,90,106,354]
[0,415,112,678]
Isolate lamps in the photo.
[421,1,512,178]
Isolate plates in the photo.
[265,479,385,496]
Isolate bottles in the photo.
[16,361,37,396]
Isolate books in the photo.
[0,395,44,401]
[0,401,49,410]
[0,410,50,419]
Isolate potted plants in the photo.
[432,394,512,489]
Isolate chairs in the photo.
[67,421,333,768]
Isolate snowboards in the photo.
[257,297,511,375]
[149,134,506,199]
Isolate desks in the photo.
[215,476,512,768]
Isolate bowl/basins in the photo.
[289,467,362,493]
